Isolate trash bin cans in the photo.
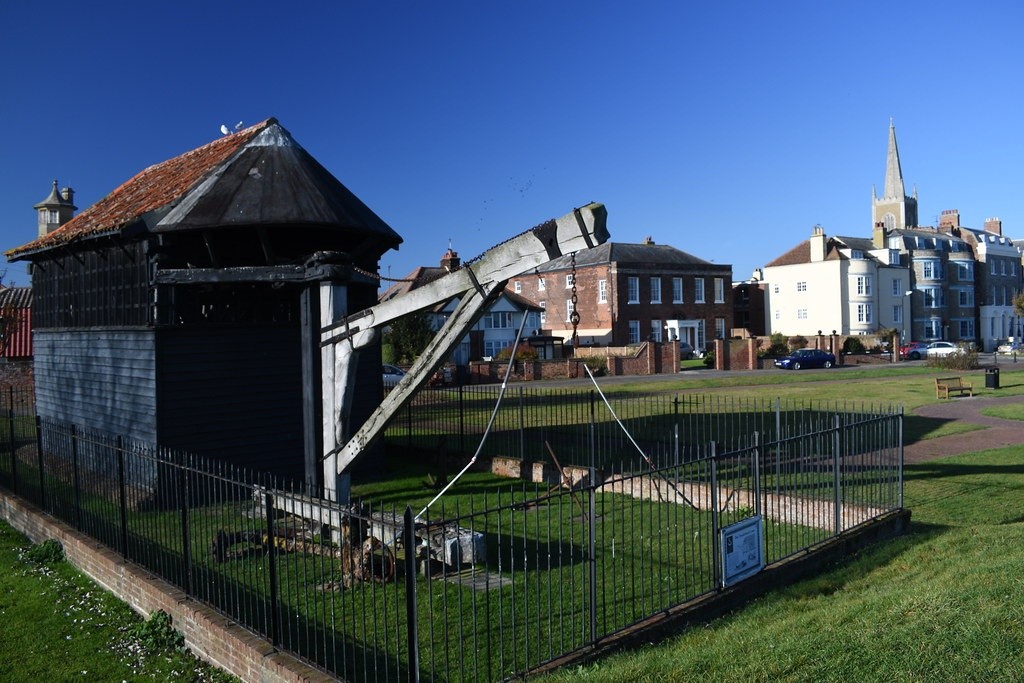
[985,367,1000,389]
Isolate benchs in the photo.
[935,376,973,399]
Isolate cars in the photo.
[680,342,694,360]
[891,342,968,360]
[382,364,406,390]
[774,348,836,370]
[997,342,1021,353]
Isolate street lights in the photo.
[902,291,913,362]
